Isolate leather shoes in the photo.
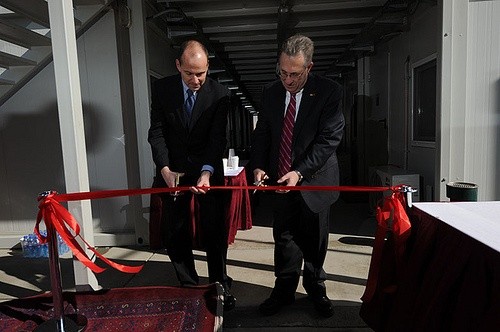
[307,294,333,309]
[262,296,296,312]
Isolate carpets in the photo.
[0,280,225,332]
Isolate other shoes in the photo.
[223,290,237,306]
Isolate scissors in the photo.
[253,172,269,194]
[170,172,185,207]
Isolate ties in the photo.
[278,93,297,180]
[186,89,195,122]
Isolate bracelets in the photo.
[294,170,303,182]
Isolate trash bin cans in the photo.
[446,182,478,202]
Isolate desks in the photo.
[412,200,500,332]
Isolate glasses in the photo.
[281,67,306,79]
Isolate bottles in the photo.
[21,230,70,260]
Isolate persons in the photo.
[148,40,236,311]
[248,35,345,319]
